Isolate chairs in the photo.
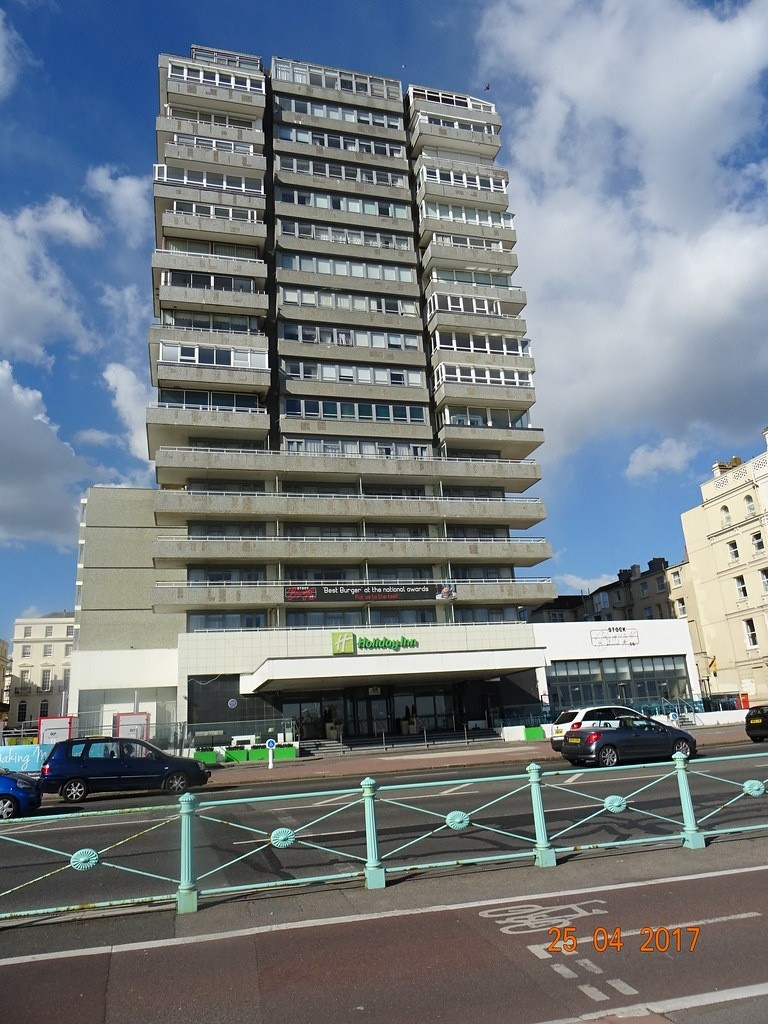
[124,745,134,757]
[110,751,115,758]
[620,720,625,727]
[604,723,611,728]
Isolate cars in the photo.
[562,715,698,767]
[550,706,654,754]
[745,704,768,742]
[0,767,42,819]
[37,735,211,803]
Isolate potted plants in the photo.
[249,744,296,761]
[524,723,545,741]
[194,748,216,763]
[323,708,343,740]
[225,745,248,762]
[400,704,420,735]
